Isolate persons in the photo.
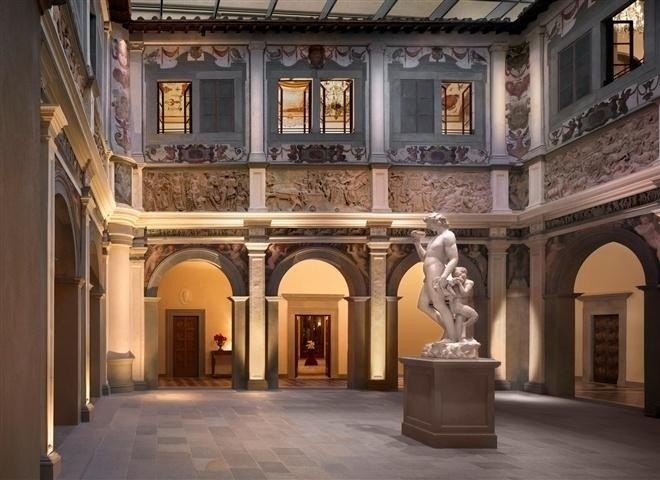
[409,213,479,340]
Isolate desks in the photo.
[210,351,233,378]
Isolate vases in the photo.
[218,340,224,352]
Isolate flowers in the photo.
[214,333,227,342]
